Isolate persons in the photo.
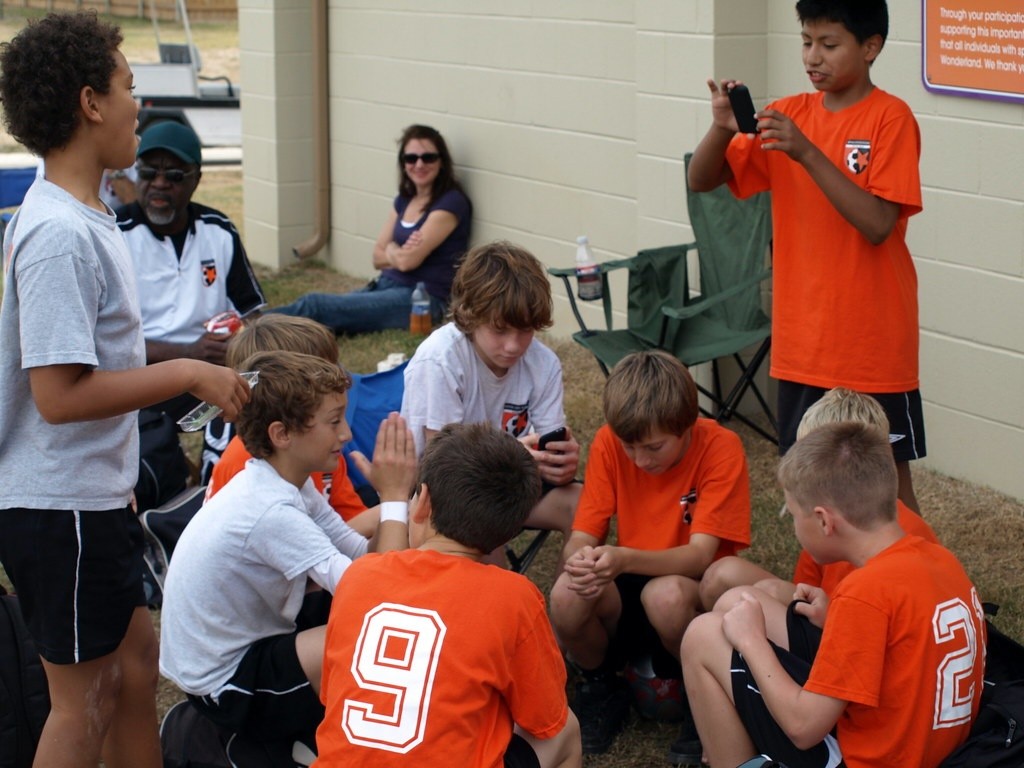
[3,16,251,765]
[109,119,267,507]
[260,124,474,336]
[549,350,753,755]
[401,243,582,585]
[156,310,416,750]
[312,423,582,768]
[686,0,924,521]
[680,384,986,767]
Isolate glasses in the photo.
[136,162,197,183]
[404,152,442,164]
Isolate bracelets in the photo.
[379,500,408,525]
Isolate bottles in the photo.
[409,281,432,336]
[574,236,603,299]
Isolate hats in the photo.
[136,120,202,167]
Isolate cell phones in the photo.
[539,427,567,452]
[728,85,761,134]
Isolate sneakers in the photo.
[669,713,704,764]
[568,692,632,755]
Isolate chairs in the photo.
[548,154,781,448]
[338,361,552,578]
